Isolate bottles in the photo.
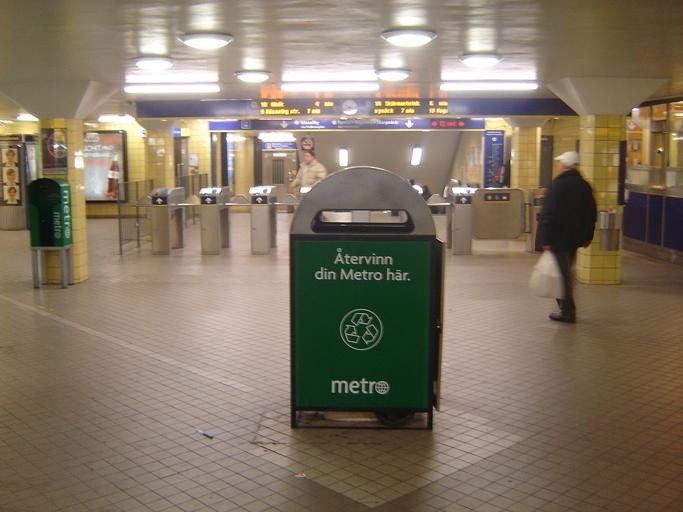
[106,159,119,198]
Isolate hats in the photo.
[554,152,579,169]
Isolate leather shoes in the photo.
[549,313,575,323]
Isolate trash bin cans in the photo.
[26,178,75,288]
[288,166,446,430]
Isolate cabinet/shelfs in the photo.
[626,108,683,169]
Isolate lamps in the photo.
[136,57,175,71]
[233,69,271,83]
[459,53,504,70]
[381,30,438,48]
[374,68,412,81]
[178,34,234,50]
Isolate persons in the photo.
[409,177,432,201]
[534,150,597,323]
[290,149,327,195]
